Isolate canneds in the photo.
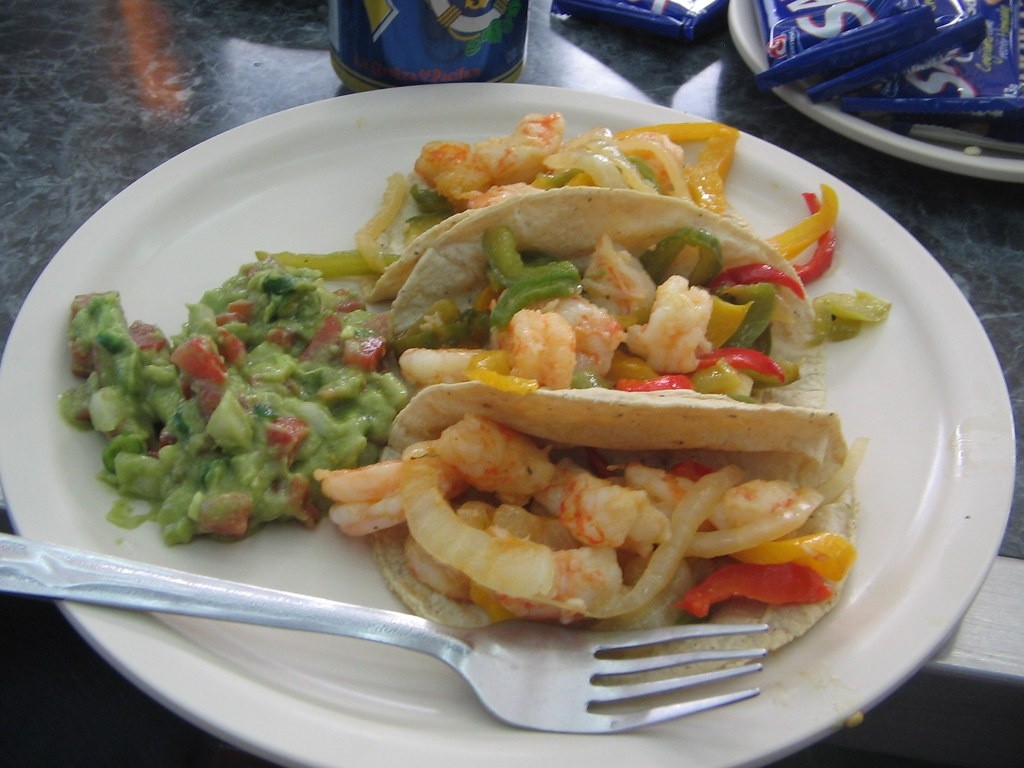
[328,0,529,91]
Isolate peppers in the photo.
[241,122,888,617]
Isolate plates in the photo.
[727,0,1024,184]
[0,79,1019,768]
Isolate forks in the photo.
[0,532,768,735]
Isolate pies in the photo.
[348,181,856,678]
